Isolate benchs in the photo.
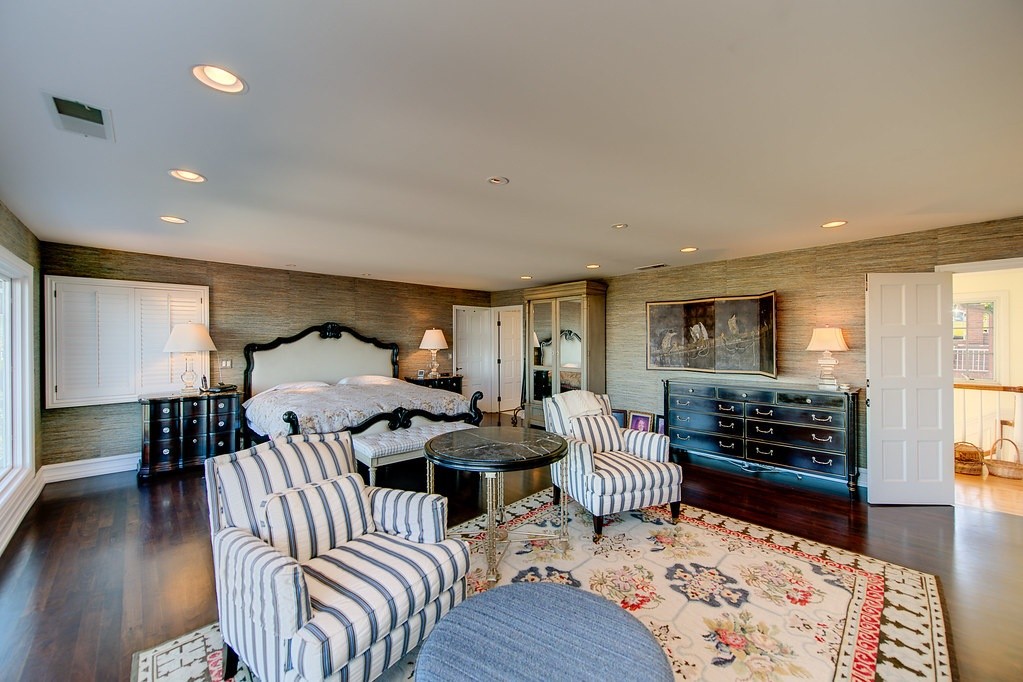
[352,422,485,488]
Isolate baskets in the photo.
[954,441,983,475]
[984,438,1023,480]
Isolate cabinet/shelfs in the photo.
[524,280,609,429]
[662,377,862,492]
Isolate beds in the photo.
[538,329,581,390]
[243,322,484,450]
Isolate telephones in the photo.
[200,373,208,392]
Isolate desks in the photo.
[423,426,568,582]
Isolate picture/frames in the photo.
[612,409,627,429]
[656,415,664,434]
[627,410,654,432]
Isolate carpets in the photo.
[130,487,957,682]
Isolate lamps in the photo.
[419,329,448,378]
[805,326,850,390]
[163,323,217,393]
[534,330,540,347]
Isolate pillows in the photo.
[569,416,626,452]
[338,375,394,385]
[276,381,332,390]
[258,473,375,564]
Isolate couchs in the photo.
[204,431,472,682]
[543,391,683,534]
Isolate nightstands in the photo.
[404,376,463,395]
[533,369,549,400]
[137,392,242,488]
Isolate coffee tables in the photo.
[414,583,676,682]
